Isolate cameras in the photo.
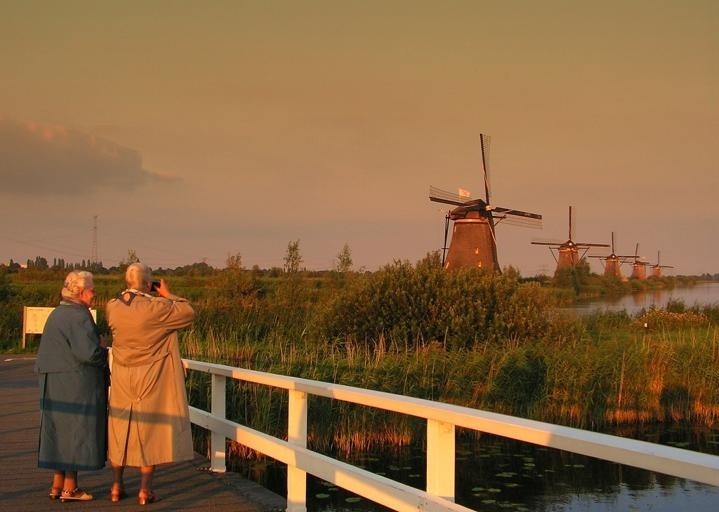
[150,280,161,292]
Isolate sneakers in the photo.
[110,479,127,502]
[137,487,163,506]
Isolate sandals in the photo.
[49,483,95,503]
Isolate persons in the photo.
[31,268,111,503]
[101,259,200,506]
